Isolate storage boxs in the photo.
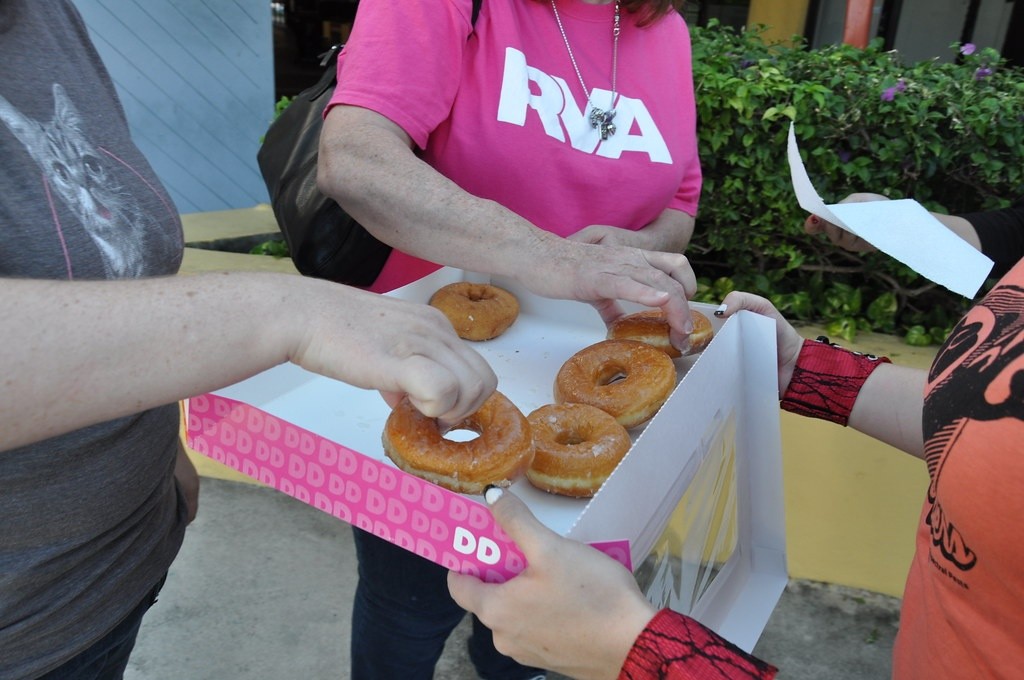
[182,264,789,653]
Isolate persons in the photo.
[806,193,1024,277]
[0,0,502,680]
[316,0,703,679]
[446,253,1024,680]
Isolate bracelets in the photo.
[618,608,779,680]
[780,336,892,428]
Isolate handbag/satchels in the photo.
[257,0,483,289]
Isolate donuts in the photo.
[381,384,537,498]
[524,402,634,499]
[553,339,678,429]
[605,307,715,360]
[430,281,519,341]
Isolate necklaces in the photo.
[549,0,622,140]
